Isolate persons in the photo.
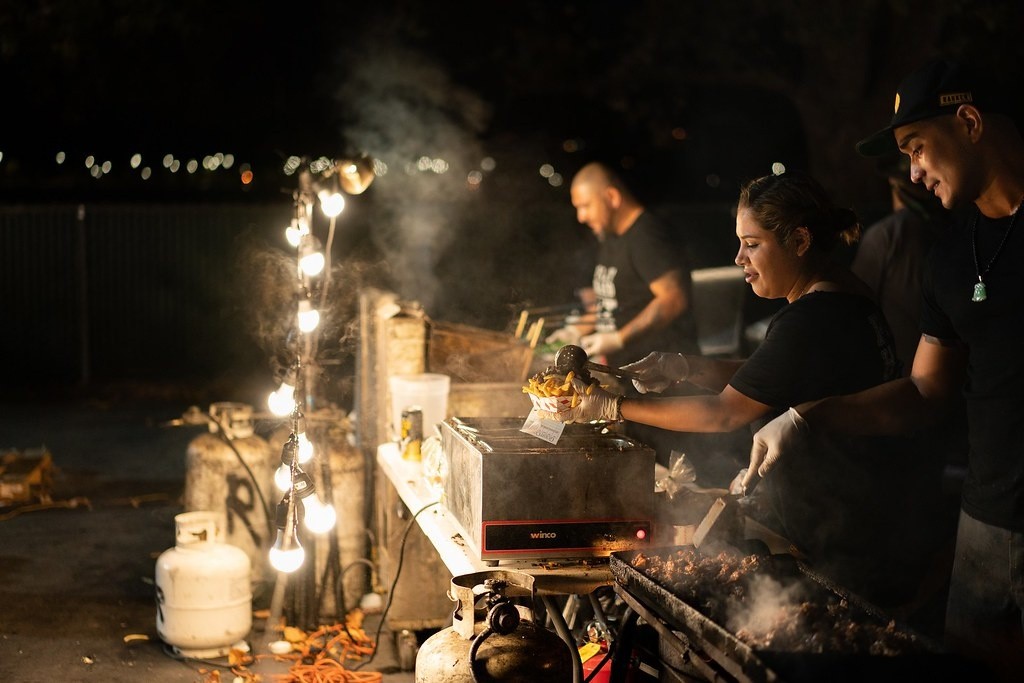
[537,173,899,434]
[546,162,702,449]
[847,160,937,377]
[741,50,1024,682]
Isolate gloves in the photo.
[741,407,810,491]
[537,377,618,424]
[581,332,622,357]
[616,351,689,394]
[544,326,580,346]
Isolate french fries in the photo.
[522,368,599,408]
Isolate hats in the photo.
[857,54,1010,157]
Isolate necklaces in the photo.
[972,197,1024,302]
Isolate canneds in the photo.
[400,406,423,464]
